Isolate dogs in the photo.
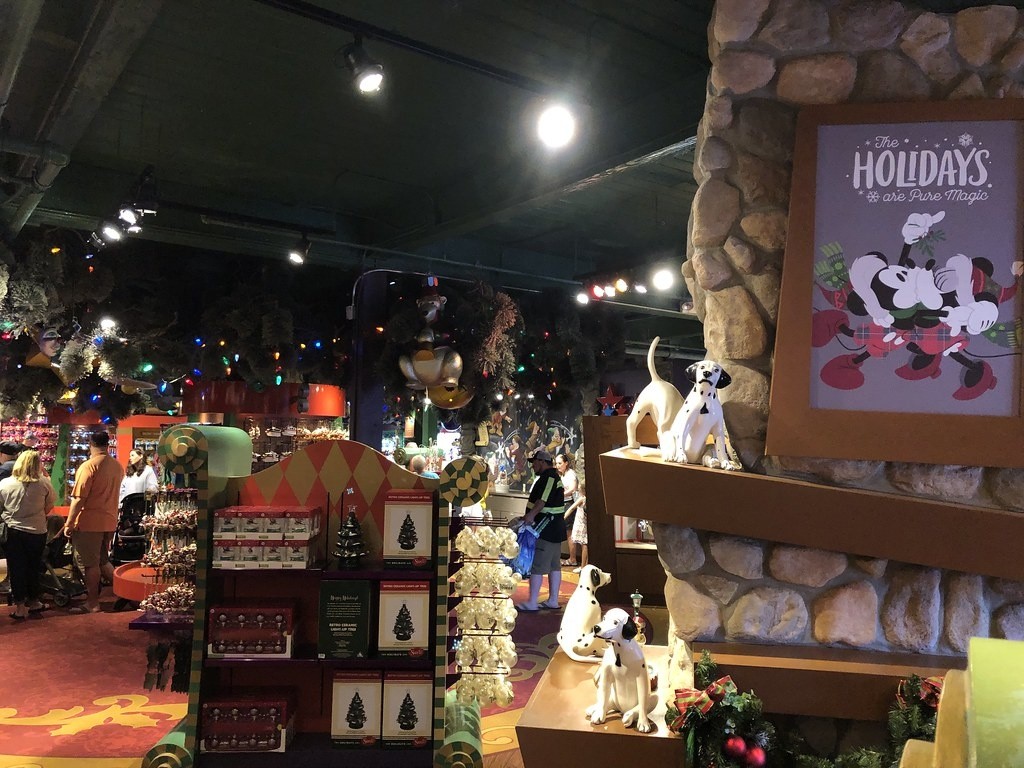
[625,336,735,472]
[585,607,658,733]
[556,564,613,664]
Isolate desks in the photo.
[513,574,691,768]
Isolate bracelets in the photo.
[64,522,75,527]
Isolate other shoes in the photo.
[113,596,130,612]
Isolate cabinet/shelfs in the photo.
[1,400,589,767]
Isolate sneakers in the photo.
[573,566,582,574]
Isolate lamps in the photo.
[123,220,144,235]
[91,229,107,247]
[332,29,386,96]
[287,231,314,265]
[126,167,161,216]
[118,200,139,226]
[576,265,679,305]
[101,213,127,241]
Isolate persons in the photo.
[63,431,132,615]
[117,447,159,522]
[0,440,18,594]
[412,454,441,480]
[17,431,42,458]
[0,449,52,621]
[513,450,566,613]
[118,504,144,536]
[555,454,579,566]
[564,480,589,574]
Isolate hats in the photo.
[526,450,553,463]
[24,434,37,440]
[0,440,20,455]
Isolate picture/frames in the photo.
[763,97,1023,468]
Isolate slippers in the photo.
[28,603,50,613]
[68,604,94,615]
[8,611,25,620]
[513,601,540,613]
[560,559,577,566]
[538,599,562,610]
